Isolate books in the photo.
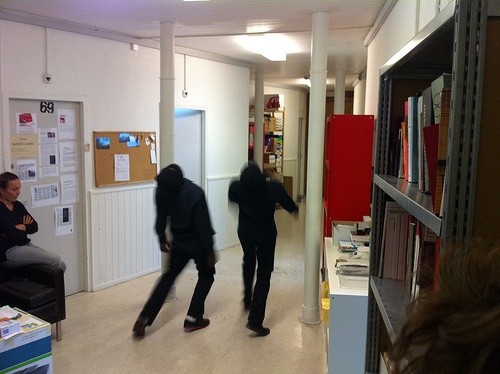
[388,70,452,301]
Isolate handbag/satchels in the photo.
[245,319,270,335]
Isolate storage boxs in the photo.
[332,220,357,245]
[0,321,19,337]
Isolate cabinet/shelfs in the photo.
[263,107,286,173]
[365,0,500,374]
[324,236,420,374]
[324,114,374,236]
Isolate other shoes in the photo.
[132,320,145,336]
[184,315,209,332]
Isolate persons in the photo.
[390,240,500,374]
[133,163,216,335]
[0,172,66,274]
[228,164,299,335]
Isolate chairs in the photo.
[0,263,66,341]
